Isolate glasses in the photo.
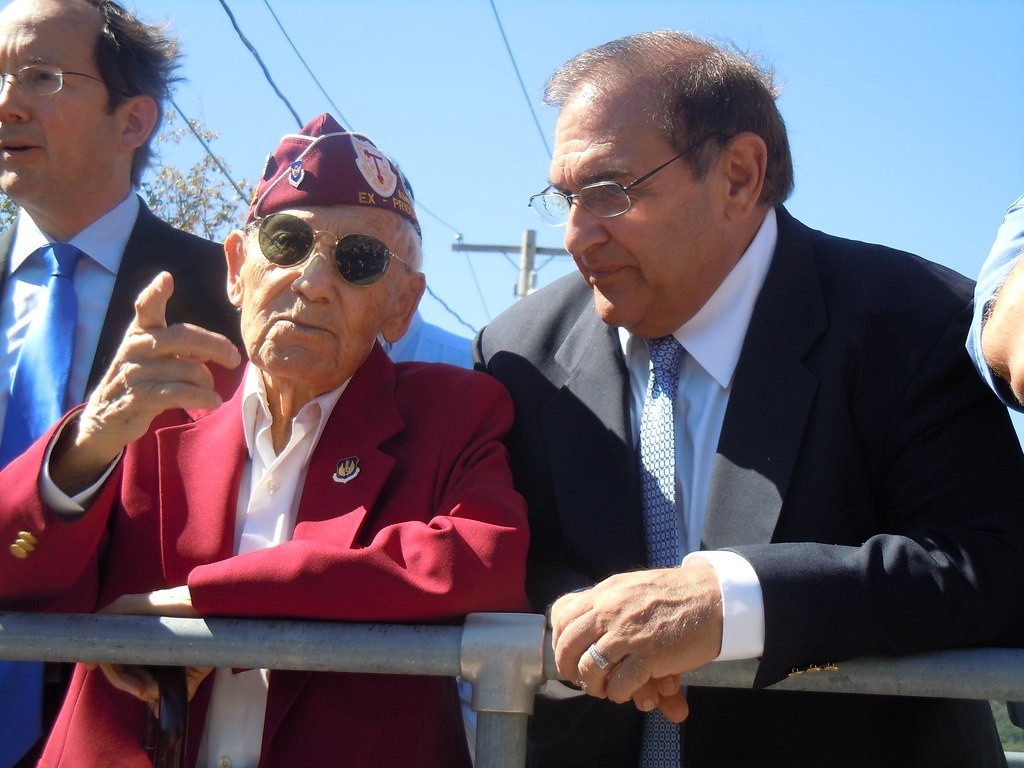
[528,130,732,228]
[0,65,136,97]
[258,213,417,287]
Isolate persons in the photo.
[460,30,1023,767]
[0,110,531,768]
[1,0,249,768]
[963,196,1023,413]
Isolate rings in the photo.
[589,644,615,672]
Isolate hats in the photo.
[244,112,422,247]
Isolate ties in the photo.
[0,243,79,768]
[635,334,687,768]
